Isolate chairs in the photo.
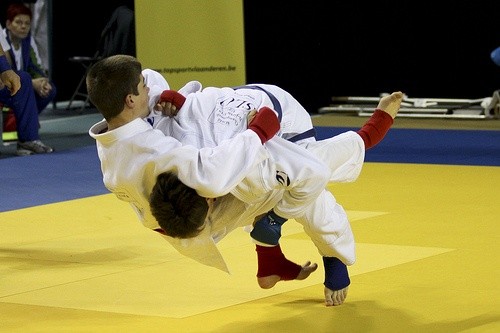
[65,7,134,114]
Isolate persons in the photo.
[0,9,54,155]
[140,69,355,306]
[86,55,402,289]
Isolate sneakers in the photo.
[16,141,53,156]
